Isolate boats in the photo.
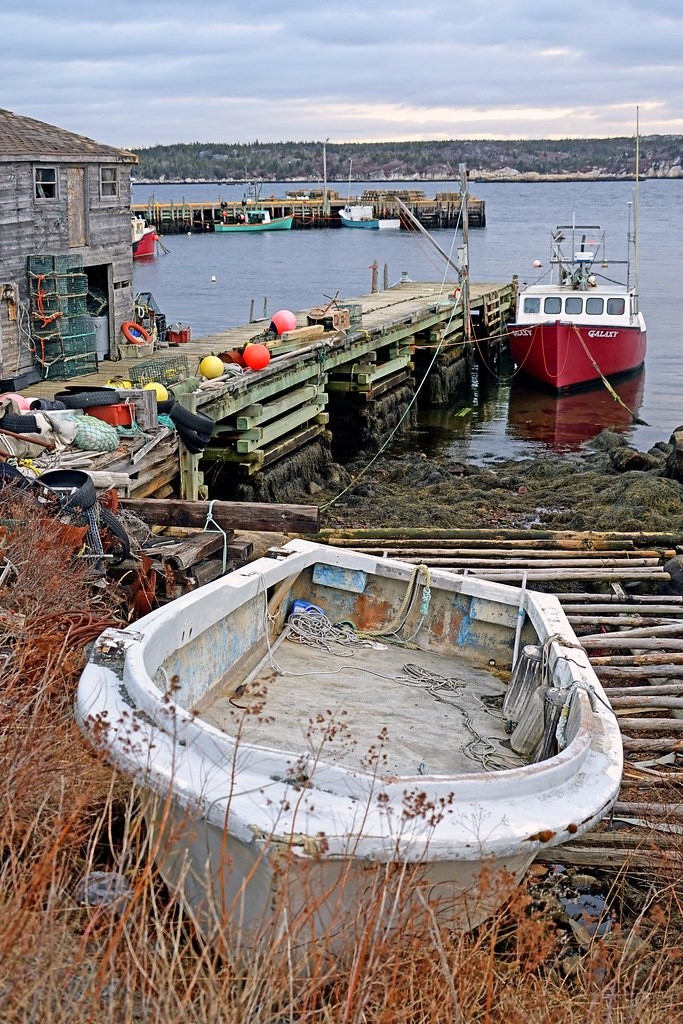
[508,105,647,396]
[214,192,294,231]
[75,540,624,993]
[132,215,158,257]
[338,158,401,228]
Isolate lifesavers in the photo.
[120,321,152,345]
[157,394,175,414]
[0,412,42,434]
[53,389,122,411]
[171,401,218,455]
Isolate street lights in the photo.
[323,137,330,213]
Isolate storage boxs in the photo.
[0,370,42,392]
[86,403,136,426]
[117,390,158,429]
[167,324,191,342]
[119,343,154,358]
[135,290,167,342]
